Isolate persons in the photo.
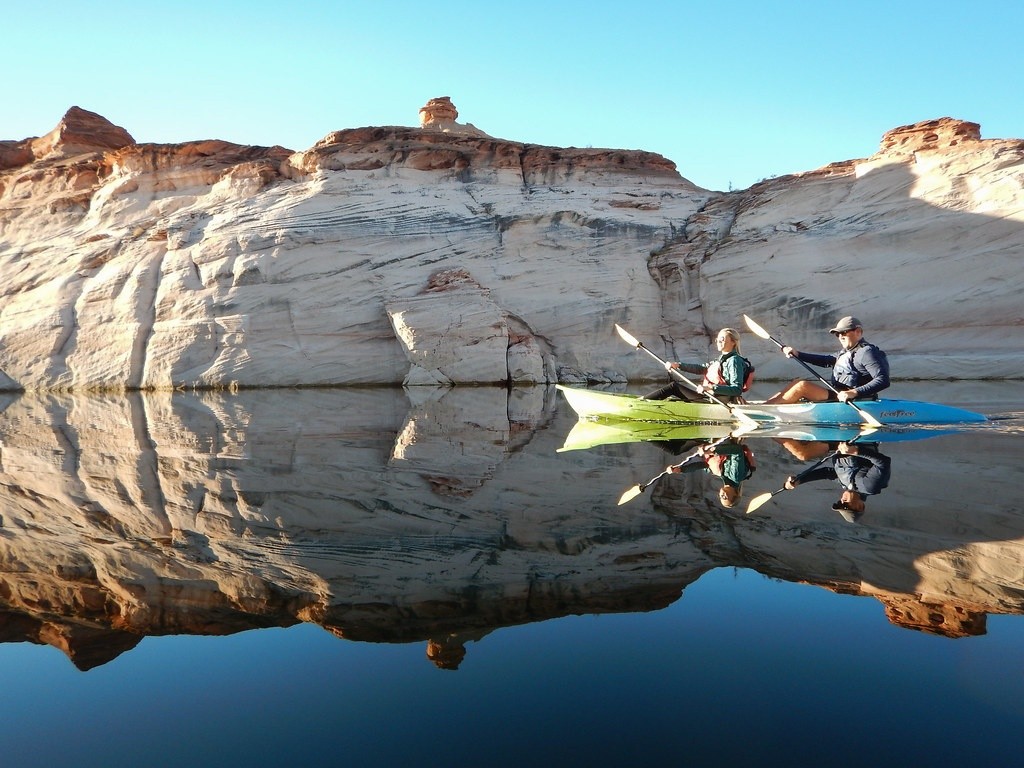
[639,328,750,404]
[765,317,891,403]
[647,438,756,508]
[773,437,891,524]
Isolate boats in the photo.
[554,424,974,455]
[555,383,993,423]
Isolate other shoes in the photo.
[737,396,748,405]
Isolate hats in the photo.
[832,503,864,524]
[829,316,862,334]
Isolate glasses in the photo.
[715,337,728,343]
[835,328,856,337]
[837,501,848,510]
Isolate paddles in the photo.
[741,313,879,426]
[745,427,880,516]
[613,324,761,430]
[616,426,760,506]
